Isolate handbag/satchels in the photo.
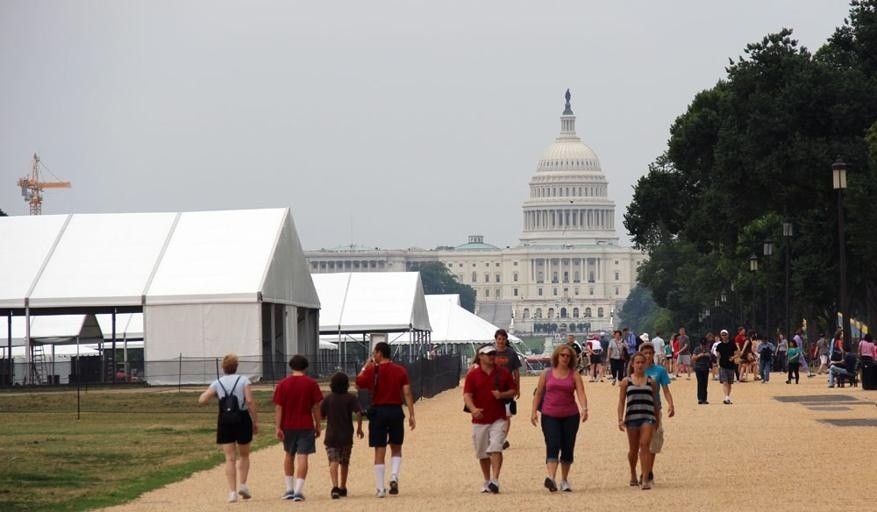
[650,427,664,454]
[496,371,512,404]
[762,348,771,363]
[358,388,372,415]
[534,388,544,412]
[831,352,842,362]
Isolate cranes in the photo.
[17,153,70,215]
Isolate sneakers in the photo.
[698,399,733,404]
[630,472,653,489]
[503,440,509,450]
[389,474,398,494]
[228,488,251,503]
[282,491,305,501]
[331,485,347,498]
[560,481,571,491]
[375,487,386,498]
[481,480,499,494]
[481,481,499,493]
[545,477,557,492]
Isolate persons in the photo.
[716,331,739,404]
[492,329,523,449]
[810,330,876,390]
[637,342,675,480]
[462,343,517,494]
[317,371,364,499]
[197,355,260,502]
[566,327,815,385]
[271,355,326,501]
[531,344,587,491]
[692,337,713,404]
[618,351,661,490]
[354,341,417,497]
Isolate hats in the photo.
[640,333,649,342]
[479,345,496,355]
[586,331,604,340]
[721,329,728,334]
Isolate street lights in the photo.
[749,251,761,332]
[697,270,744,342]
[761,233,776,342]
[831,152,850,353]
[780,210,793,341]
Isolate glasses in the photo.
[481,351,496,356]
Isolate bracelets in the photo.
[582,408,588,411]
[362,368,366,372]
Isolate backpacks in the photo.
[218,375,241,414]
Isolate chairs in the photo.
[836,357,860,386]
